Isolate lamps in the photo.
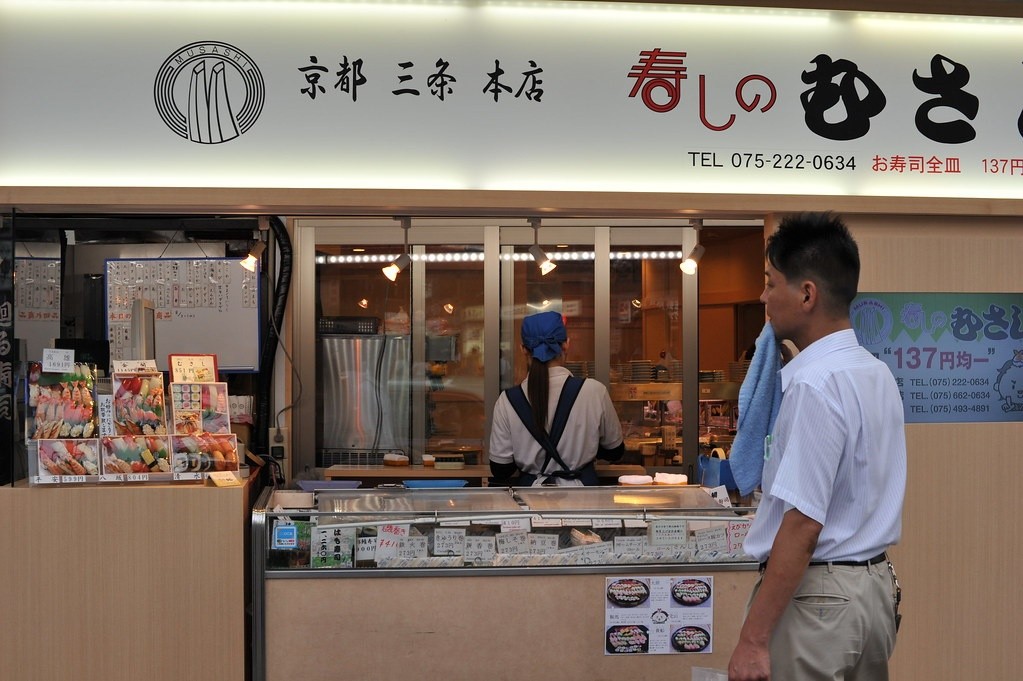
[679,217,708,276]
[383,215,415,282]
[526,216,558,276]
[239,216,275,273]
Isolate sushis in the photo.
[102,374,170,476]
[671,626,710,652]
[672,579,711,606]
[608,579,650,607]
[26,361,97,476]
[605,626,648,654]
[173,385,238,474]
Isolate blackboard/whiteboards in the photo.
[103,258,262,374]
[13,257,60,341]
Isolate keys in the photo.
[888,561,903,632]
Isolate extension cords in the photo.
[268,427,288,488]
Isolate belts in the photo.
[807,549,886,569]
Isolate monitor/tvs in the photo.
[54,338,109,375]
[132,298,156,362]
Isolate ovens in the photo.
[322,332,411,468]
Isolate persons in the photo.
[486,312,628,487]
[727,210,910,681]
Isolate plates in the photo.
[565,358,751,385]
